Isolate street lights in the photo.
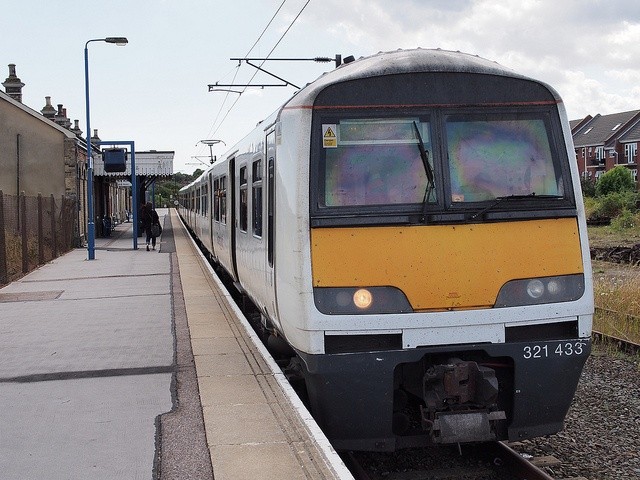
[85,37,128,260]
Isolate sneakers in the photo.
[146,244,150,250]
[152,246,156,249]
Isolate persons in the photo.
[147,201,152,210]
[141,205,162,251]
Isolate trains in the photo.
[176,49,593,453]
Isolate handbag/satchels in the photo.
[147,221,161,237]
